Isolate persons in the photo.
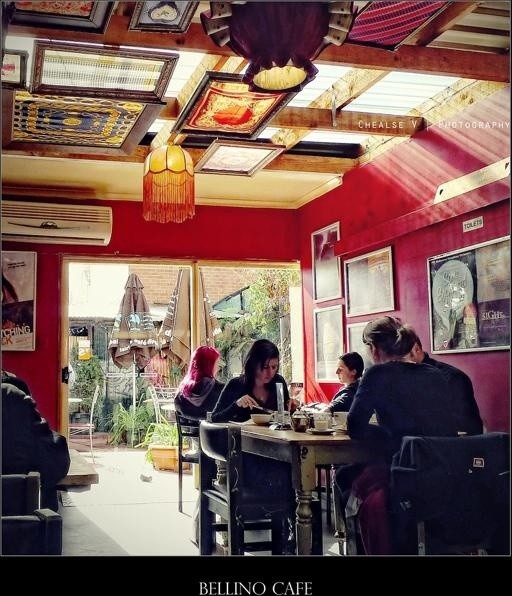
[333,315,464,541]
[406,333,483,434]
[440,302,482,349]
[173,346,226,453]
[0,369,30,397]
[1,382,71,517]
[317,351,365,528]
[212,339,300,555]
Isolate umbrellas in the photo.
[158,265,220,374]
[107,273,162,447]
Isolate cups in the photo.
[271,410,349,432]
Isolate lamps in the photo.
[199,0,358,94]
[142,144,195,223]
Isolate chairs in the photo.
[67,385,100,466]
[147,385,178,425]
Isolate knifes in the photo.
[251,403,273,413]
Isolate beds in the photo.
[68,397,82,403]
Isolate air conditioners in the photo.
[0,200,113,247]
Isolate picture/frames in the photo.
[313,304,343,384]
[1,0,197,158]
[347,322,375,376]
[427,234,512,355]
[310,221,343,305]
[0,251,37,352]
[193,141,286,177]
[170,71,299,141]
[344,246,396,317]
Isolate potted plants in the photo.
[69,342,106,434]
[102,401,154,449]
[135,420,191,471]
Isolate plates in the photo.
[432,260,474,322]
[307,428,336,434]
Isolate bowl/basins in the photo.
[251,414,271,425]
